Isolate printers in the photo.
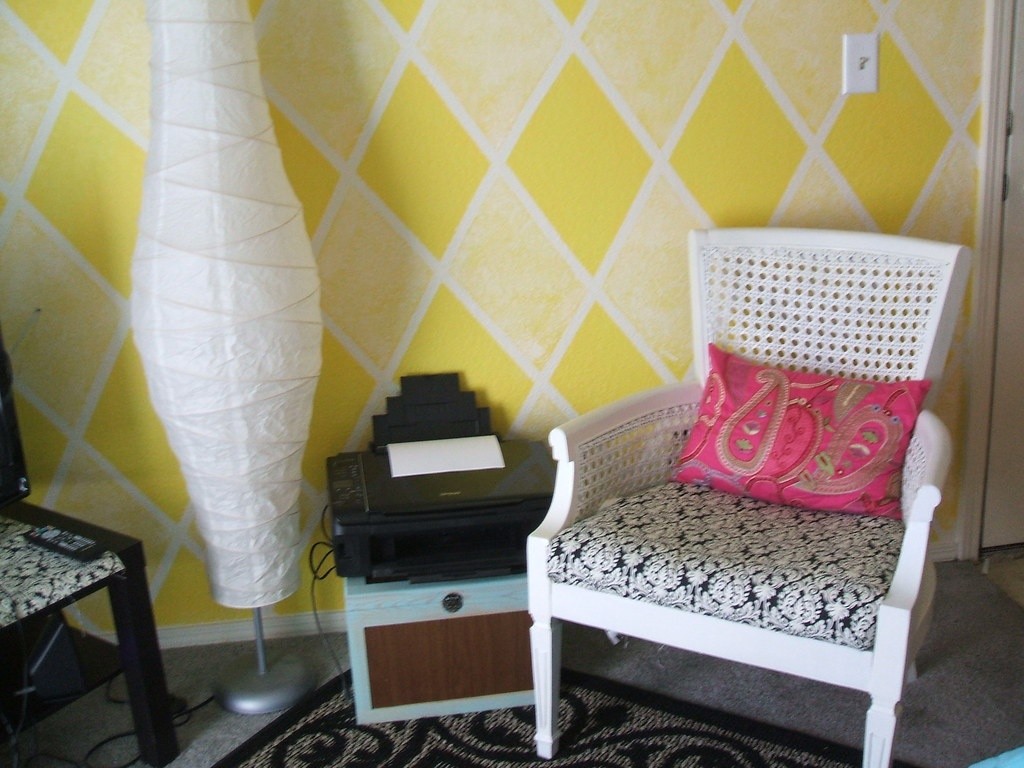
[327,373,558,584]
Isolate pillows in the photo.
[672,342,932,520]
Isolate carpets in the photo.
[209,667,931,768]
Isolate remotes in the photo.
[22,524,106,563]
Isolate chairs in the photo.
[525,224,974,768]
[0,335,183,768]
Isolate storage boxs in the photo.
[342,575,538,728]
